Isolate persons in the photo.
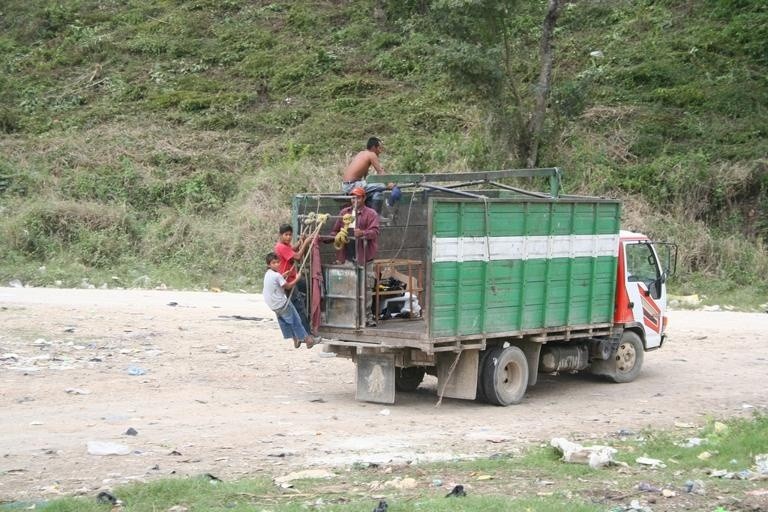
[341,136,390,223]
[261,252,321,349]
[273,224,314,338]
[322,188,380,327]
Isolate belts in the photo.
[343,180,360,184]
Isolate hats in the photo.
[350,188,365,196]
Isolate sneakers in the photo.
[294,340,301,348]
[366,315,376,327]
[307,336,321,348]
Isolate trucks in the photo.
[291,167,678,405]
